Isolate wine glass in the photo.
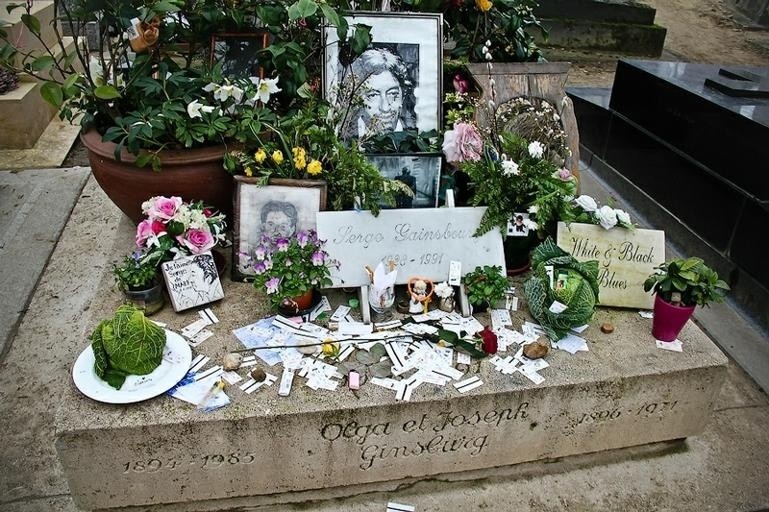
[367,284,396,323]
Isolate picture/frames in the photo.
[208,27,269,85]
[230,175,329,283]
[320,7,446,155]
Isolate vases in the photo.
[74,116,276,227]
[276,289,319,311]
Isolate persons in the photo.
[177,254,221,308]
[338,47,416,148]
[251,202,302,273]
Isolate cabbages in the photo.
[92,304,166,390]
[524,235,600,342]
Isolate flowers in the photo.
[449,3,552,63]
[132,194,231,262]
[236,227,344,311]
[230,324,500,359]
[0,1,374,174]
[447,37,639,242]
[220,109,417,217]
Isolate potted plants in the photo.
[641,255,733,342]
[110,257,167,316]
[462,259,509,315]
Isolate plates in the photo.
[73,328,192,404]
